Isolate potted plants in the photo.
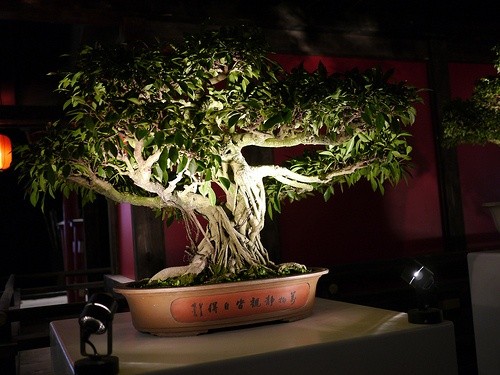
[440,47,500,239]
[9,23,432,338]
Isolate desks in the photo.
[49,294,458,375]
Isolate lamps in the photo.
[403,263,442,327]
[73,289,119,374]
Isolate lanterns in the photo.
[0,132,13,171]
